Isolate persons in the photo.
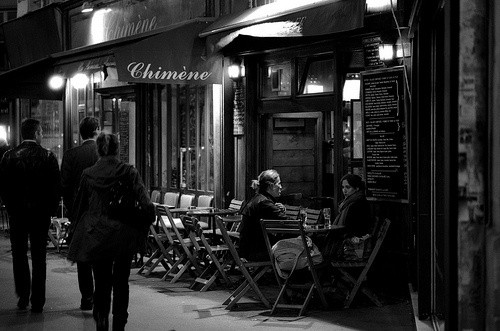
[60,117,102,310]
[324,174,376,256]
[238,169,299,262]
[68,134,155,331]
[0,119,60,314]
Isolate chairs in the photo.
[331,217,393,309]
[136,189,325,319]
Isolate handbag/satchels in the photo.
[342,234,370,262]
[106,256,130,278]
[271,234,323,280]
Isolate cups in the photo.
[323,208,331,225]
[299,207,307,227]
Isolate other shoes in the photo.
[18,297,29,309]
[80,303,93,310]
[31,307,43,313]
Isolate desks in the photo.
[192,209,240,244]
[307,224,344,232]
[168,206,210,212]
